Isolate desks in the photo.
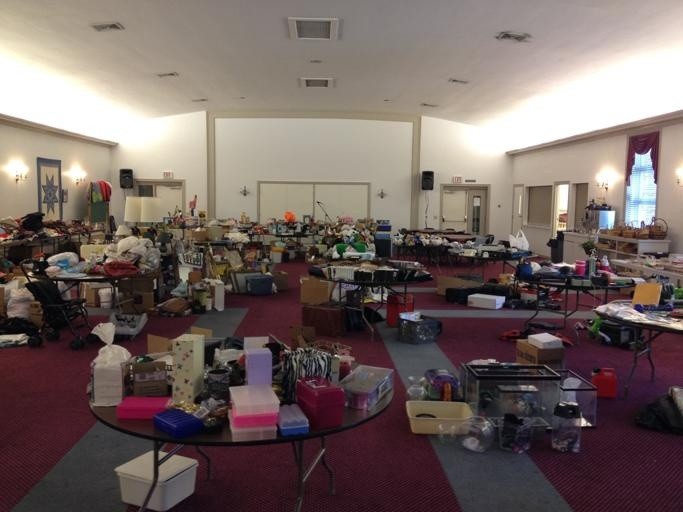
[591,299,683,397]
[399,228,530,282]
[1,228,107,268]
[193,240,239,281]
[601,257,682,305]
[307,264,431,342]
[24,269,153,332]
[255,230,315,260]
[519,277,636,335]
[84,352,398,511]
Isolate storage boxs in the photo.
[298,275,334,307]
[435,273,507,310]
[77,281,114,308]
[129,360,169,396]
[113,449,201,512]
[183,227,208,245]
[516,331,565,373]
[148,324,213,354]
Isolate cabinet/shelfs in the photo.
[596,235,670,270]
[562,232,596,265]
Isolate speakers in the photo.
[119,169,133,188]
[422,171,433,190]
